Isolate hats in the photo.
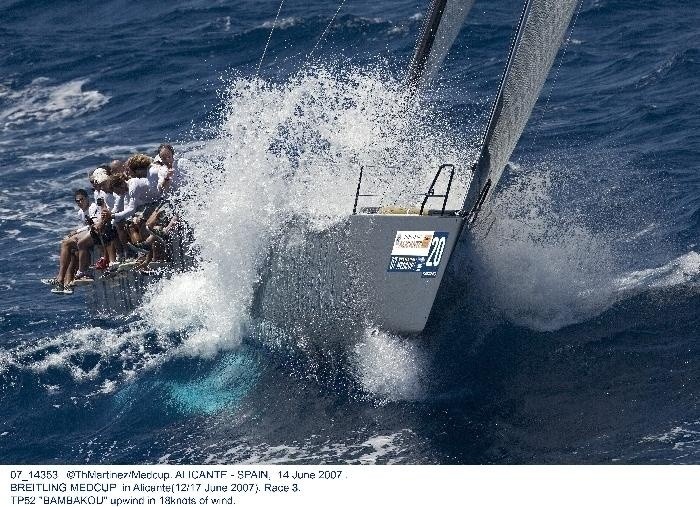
[93,168,108,184]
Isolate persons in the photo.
[40,145,196,294]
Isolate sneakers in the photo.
[128,241,151,253]
[89,257,142,280]
[146,226,166,241]
[41,275,94,294]
